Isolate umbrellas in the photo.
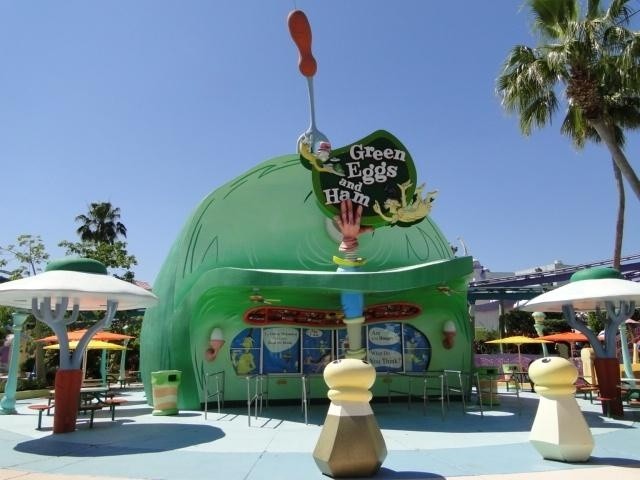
[43,339,133,387]
[534,332,590,357]
[33,327,136,380]
[485,335,555,372]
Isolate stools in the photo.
[105,399,127,421]
[28,405,52,430]
[498,373,640,417]
[80,403,104,429]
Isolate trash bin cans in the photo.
[476,366,499,405]
[150,370,182,416]
[502,364,521,389]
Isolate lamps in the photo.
[442,321,456,348]
[205,329,224,361]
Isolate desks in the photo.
[45,371,138,416]
